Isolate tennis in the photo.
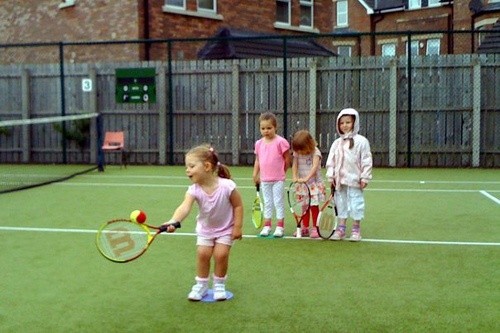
[129,209,147,224]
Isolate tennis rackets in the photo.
[287,181,312,239]
[316,179,338,240]
[251,183,265,229]
[95,219,183,263]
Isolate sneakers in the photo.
[189,284,208,300]
[214,284,226,300]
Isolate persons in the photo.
[253,112,291,237]
[161,143,244,302]
[326,108,373,242]
[291,130,327,238]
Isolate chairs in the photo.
[102,131,126,169]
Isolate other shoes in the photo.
[311,227,319,237]
[273,226,284,236]
[260,226,271,235]
[330,228,346,240]
[349,229,361,241]
[293,227,309,237]
